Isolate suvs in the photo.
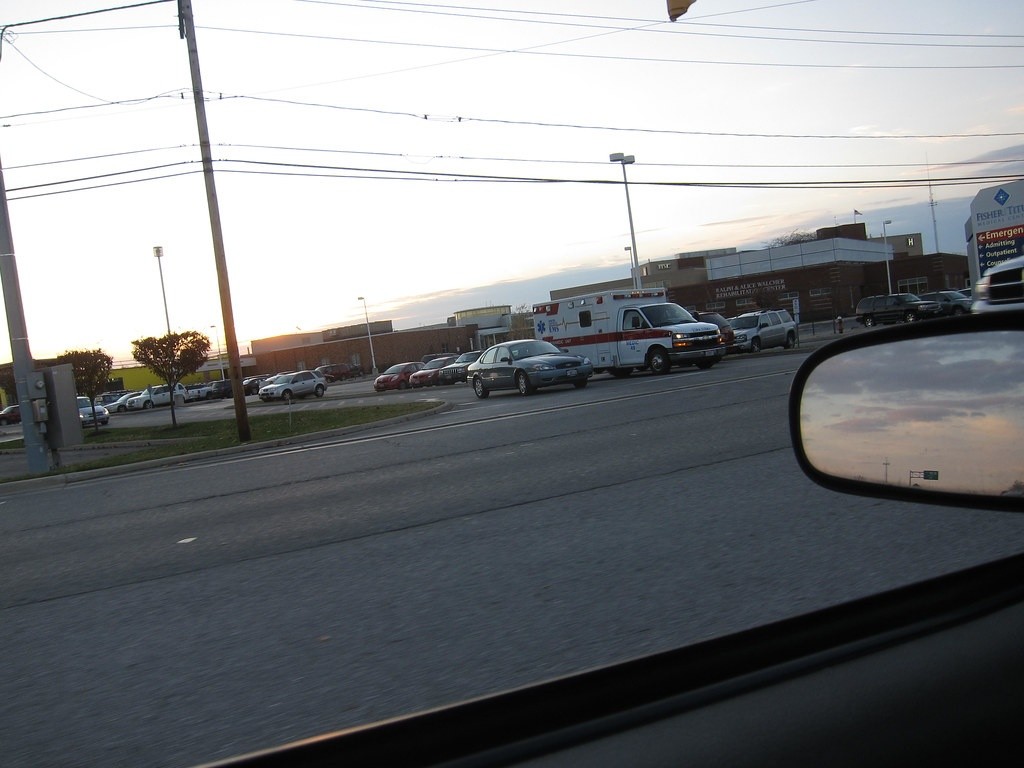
[692,311,734,349]
[125,384,189,410]
[855,294,943,328]
[730,309,796,352]
[258,370,328,401]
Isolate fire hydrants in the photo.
[836,316,844,334]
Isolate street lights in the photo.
[153,246,173,337]
[609,153,644,290]
[883,219,893,294]
[210,325,224,379]
[623,246,635,289]
[358,297,376,368]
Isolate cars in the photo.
[467,338,595,399]
[971,255,1024,313]
[185,364,363,402]
[916,291,971,317]
[0,405,21,427]
[373,362,427,392]
[409,355,461,387]
[96,390,136,405]
[420,354,460,365]
[75,396,110,428]
[439,349,487,385]
[104,392,143,413]
[958,289,974,298]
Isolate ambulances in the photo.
[531,286,725,376]
